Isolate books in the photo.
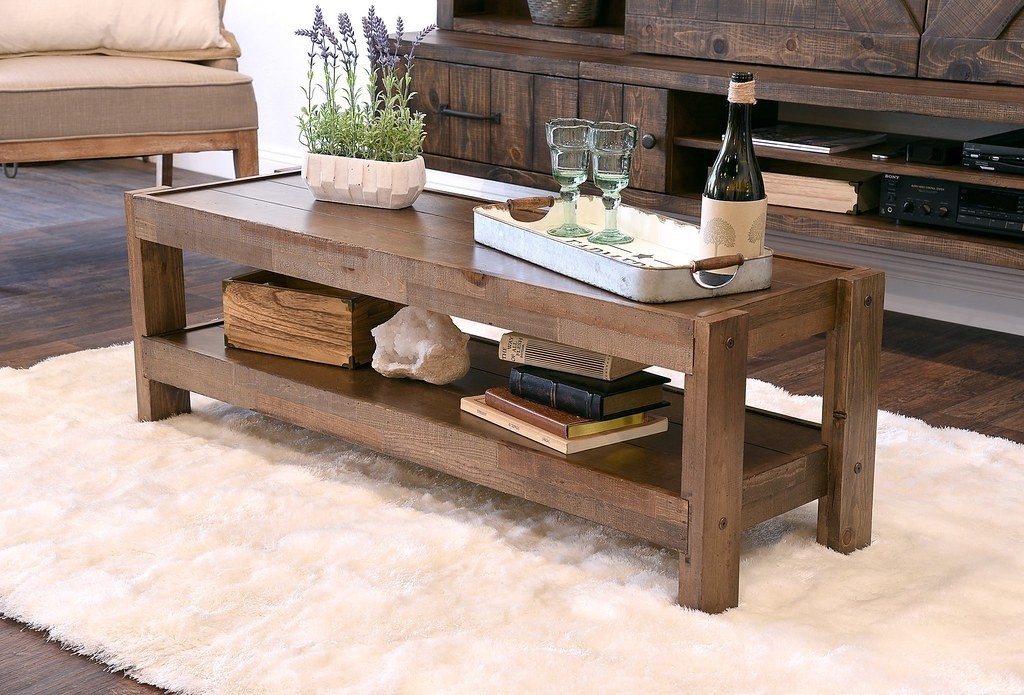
[750,123,888,155]
[462,332,671,455]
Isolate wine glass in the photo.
[588,120,639,247]
[545,118,594,238]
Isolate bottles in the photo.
[699,70,768,289]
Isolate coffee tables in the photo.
[124,165,888,615]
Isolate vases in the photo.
[302,146,427,209]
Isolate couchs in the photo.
[1,0,260,181]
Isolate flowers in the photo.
[294,4,439,161]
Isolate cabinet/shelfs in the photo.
[376,53,687,197]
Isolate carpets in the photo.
[0,314,1024,694]
